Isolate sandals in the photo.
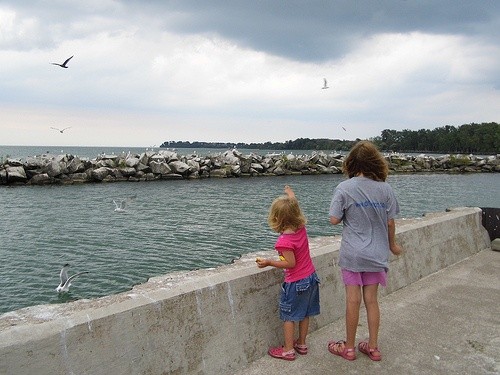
[268,345,296,360]
[358,342,381,361]
[328,340,356,360]
[294,340,308,354]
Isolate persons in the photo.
[256,185,321,361]
[327,141,401,360]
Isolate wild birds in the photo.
[56,264,89,293]
[51,126,72,133]
[112,199,126,213]
[321,78,329,89]
[50,56,73,68]
